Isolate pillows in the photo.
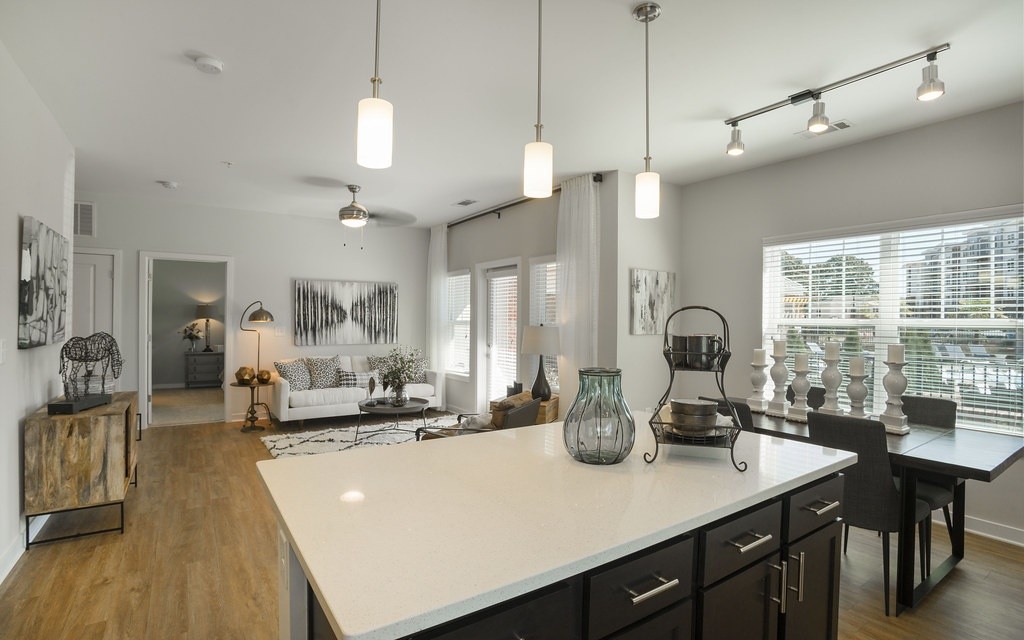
[274,358,428,391]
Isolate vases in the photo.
[560,366,635,465]
[191,341,196,352]
[388,384,410,407]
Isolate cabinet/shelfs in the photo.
[24,392,140,549]
[185,354,223,388]
[424,472,845,640]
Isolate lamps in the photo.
[727,52,943,156]
[235,301,274,426]
[195,304,219,352]
[340,0,660,228]
[521,324,561,401]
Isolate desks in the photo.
[355,397,429,441]
[717,398,1024,617]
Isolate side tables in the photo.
[229,381,275,432]
[490,391,558,427]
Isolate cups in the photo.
[672,333,723,371]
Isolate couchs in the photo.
[267,357,443,428]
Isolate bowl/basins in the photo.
[669,398,719,436]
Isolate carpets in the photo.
[260,414,468,459]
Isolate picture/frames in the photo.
[18,216,70,349]
[630,268,676,336]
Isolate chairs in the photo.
[415,397,543,441]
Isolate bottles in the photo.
[561,367,636,464]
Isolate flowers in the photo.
[368,343,428,387]
[177,323,204,341]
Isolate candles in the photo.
[754,339,905,376]
